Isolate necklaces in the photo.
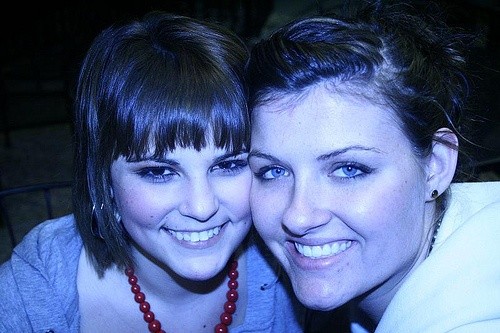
[431,208,445,257]
[124,244,240,333]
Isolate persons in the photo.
[1,13,308,333]
[238,13,500,333]
